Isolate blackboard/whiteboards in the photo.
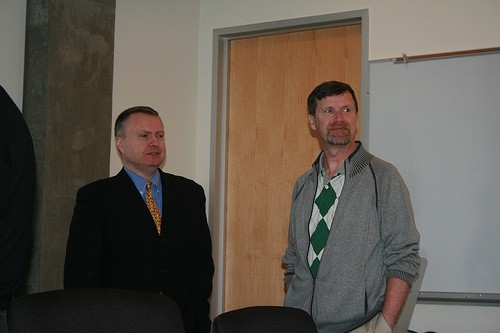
[368,47,496,303]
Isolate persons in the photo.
[282,81,420,333]
[63,106,215,333]
[0,84,36,333]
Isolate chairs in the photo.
[7,289,184,333]
[212,306,318,333]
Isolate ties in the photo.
[146,180,162,236]
